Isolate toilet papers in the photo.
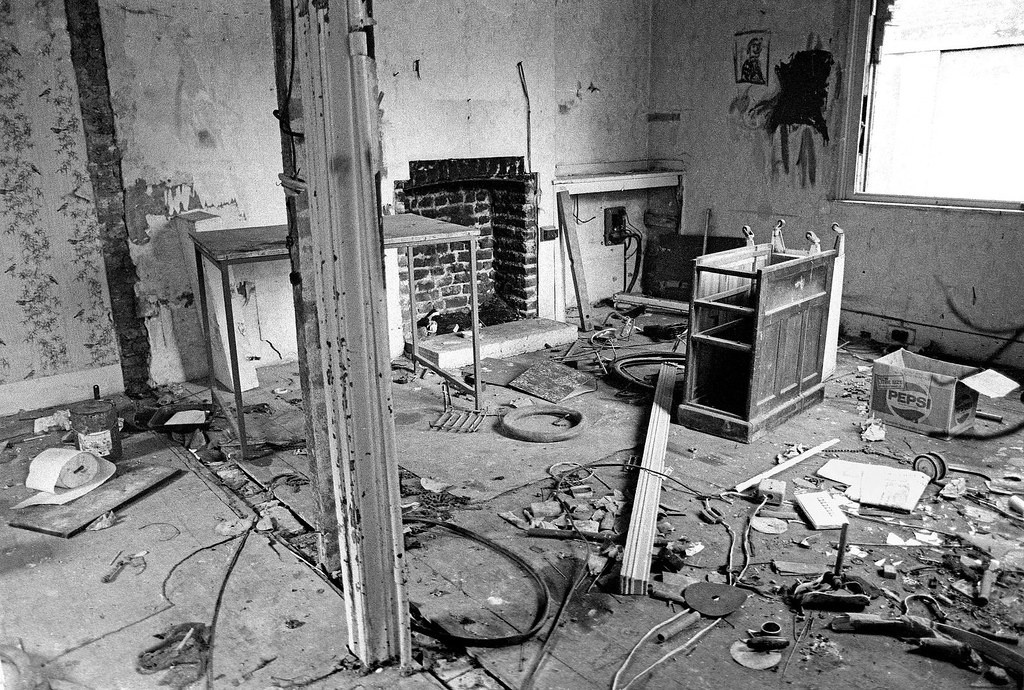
[10,446,118,512]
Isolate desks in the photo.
[189,213,481,461]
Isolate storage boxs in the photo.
[870,348,1021,442]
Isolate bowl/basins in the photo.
[147,404,218,433]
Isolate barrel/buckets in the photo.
[69,401,123,464]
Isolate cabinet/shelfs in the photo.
[677,220,845,444]
